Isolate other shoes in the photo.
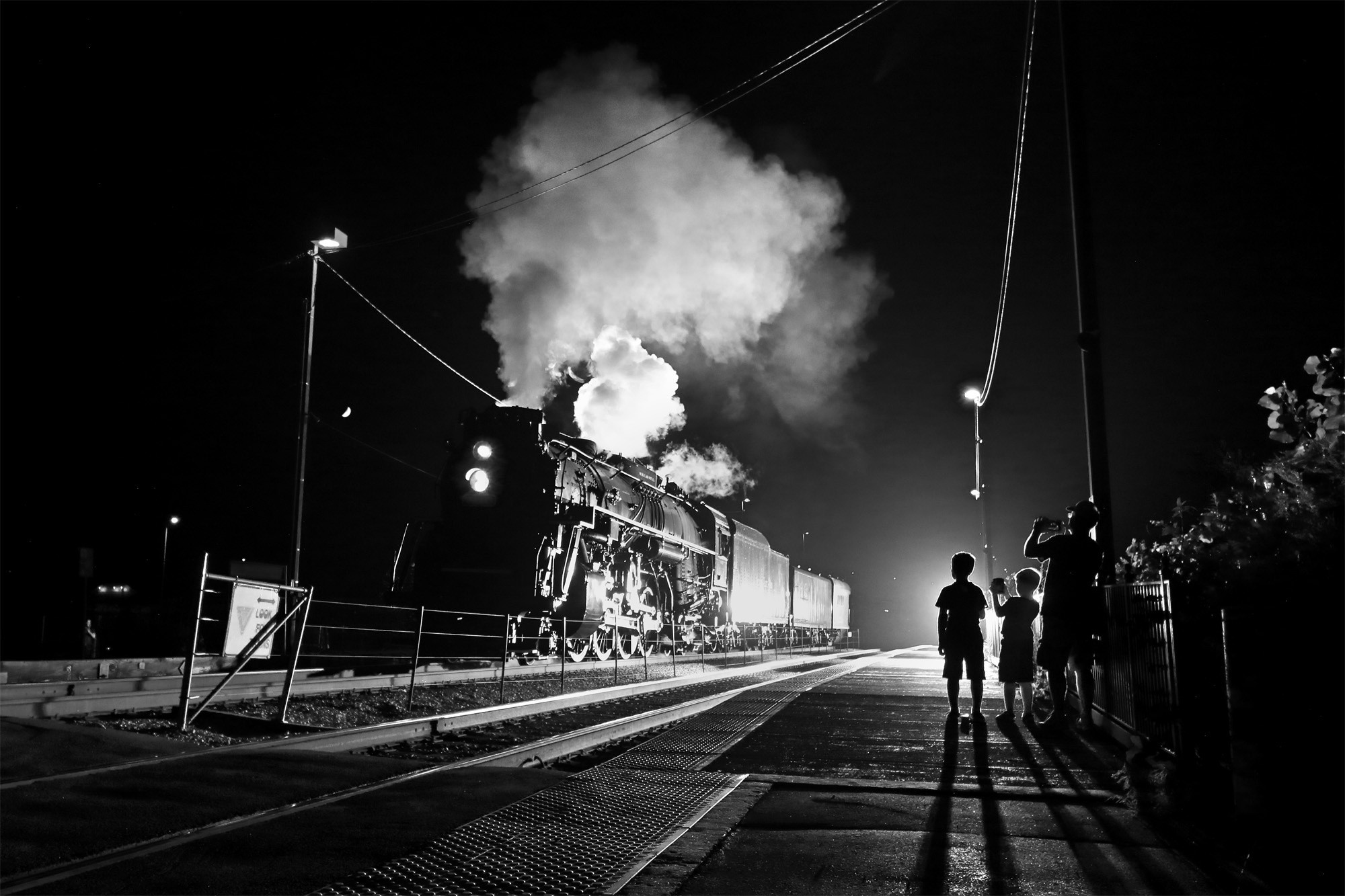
[996,711,1015,723]
[947,709,960,719]
[1038,709,1070,730]
[1076,716,1095,729]
[1021,713,1033,722]
[972,711,980,719]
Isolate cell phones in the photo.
[1048,521,1061,530]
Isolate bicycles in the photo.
[1030,638,1070,728]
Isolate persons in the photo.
[80,619,95,660]
[935,553,986,721]
[1024,500,1105,730]
[989,568,1041,721]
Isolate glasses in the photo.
[1067,512,1079,518]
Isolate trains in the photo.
[318,401,853,677]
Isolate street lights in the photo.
[965,389,994,587]
[291,224,348,651]
[163,516,178,567]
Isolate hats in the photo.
[1066,500,1099,527]
